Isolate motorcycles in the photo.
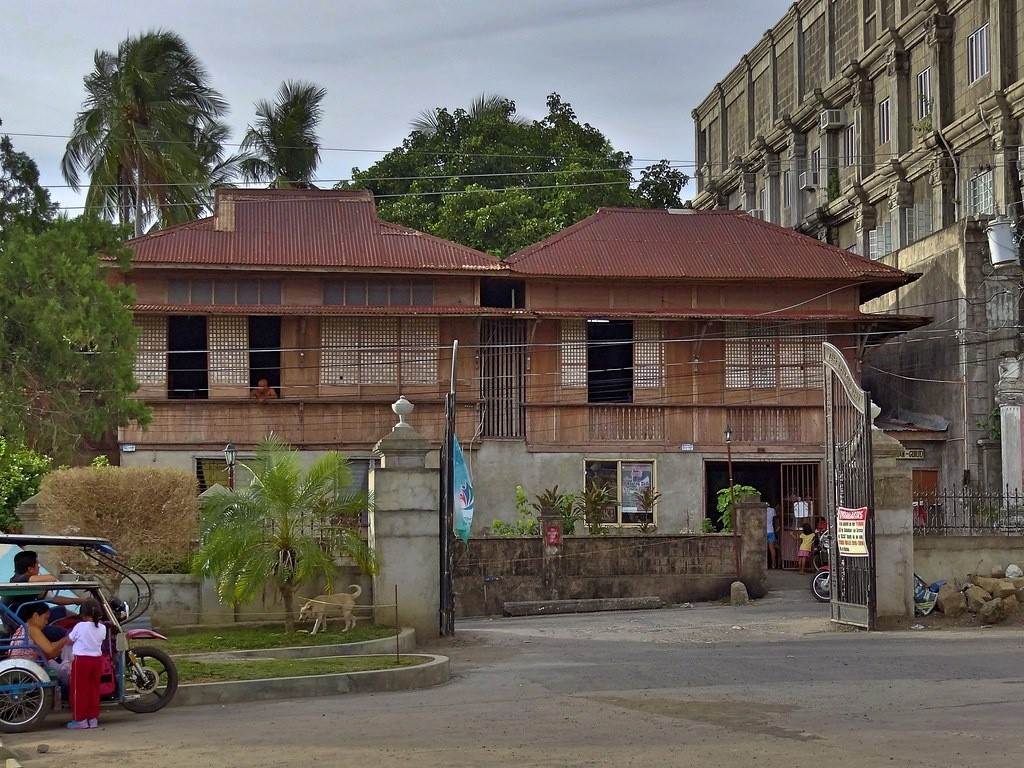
[0,597,179,732]
[810,561,844,603]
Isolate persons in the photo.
[0,551,85,643]
[250,379,278,399]
[817,517,827,529]
[766,503,778,569]
[8,603,70,699]
[67,599,107,729]
[789,523,816,574]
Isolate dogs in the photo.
[298,584,362,636]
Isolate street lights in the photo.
[223,440,238,492]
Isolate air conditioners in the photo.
[798,171,818,191]
[748,208,762,219]
[821,109,845,129]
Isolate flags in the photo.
[453,435,473,544]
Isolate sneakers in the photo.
[67,717,98,729]
